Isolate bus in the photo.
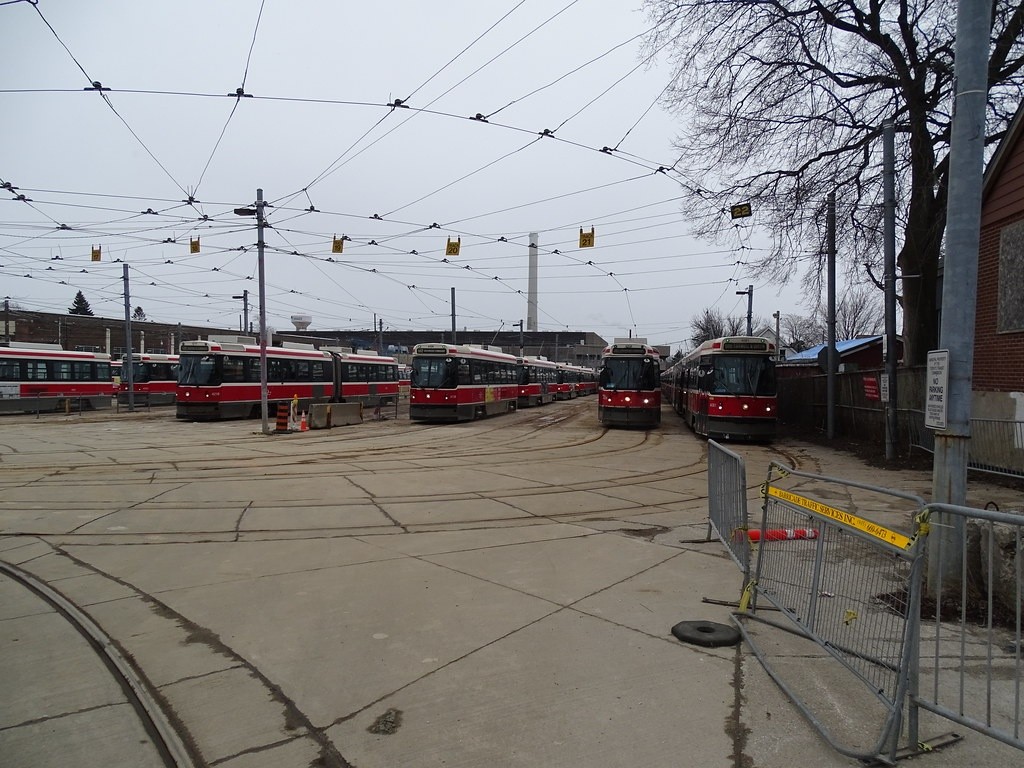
[659,359,682,417]
[477,363,556,384]
[176,339,400,421]
[592,369,601,393]
[683,336,777,443]
[578,368,595,397]
[388,364,438,398]
[515,355,556,408]
[86,360,123,396]
[404,343,519,421]
[0,340,115,411]
[117,352,180,405]
[555,362,578,400]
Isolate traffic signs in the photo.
[881,373,890,403]
[926,349,949,431]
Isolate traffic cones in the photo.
[371,407,381,421]
[297,410,309,431]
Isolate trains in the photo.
[594,343,660,430]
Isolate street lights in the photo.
[735,285,754,336]
[512,319,524,358]
[233,188,270,431]
[232,290,248,336]
